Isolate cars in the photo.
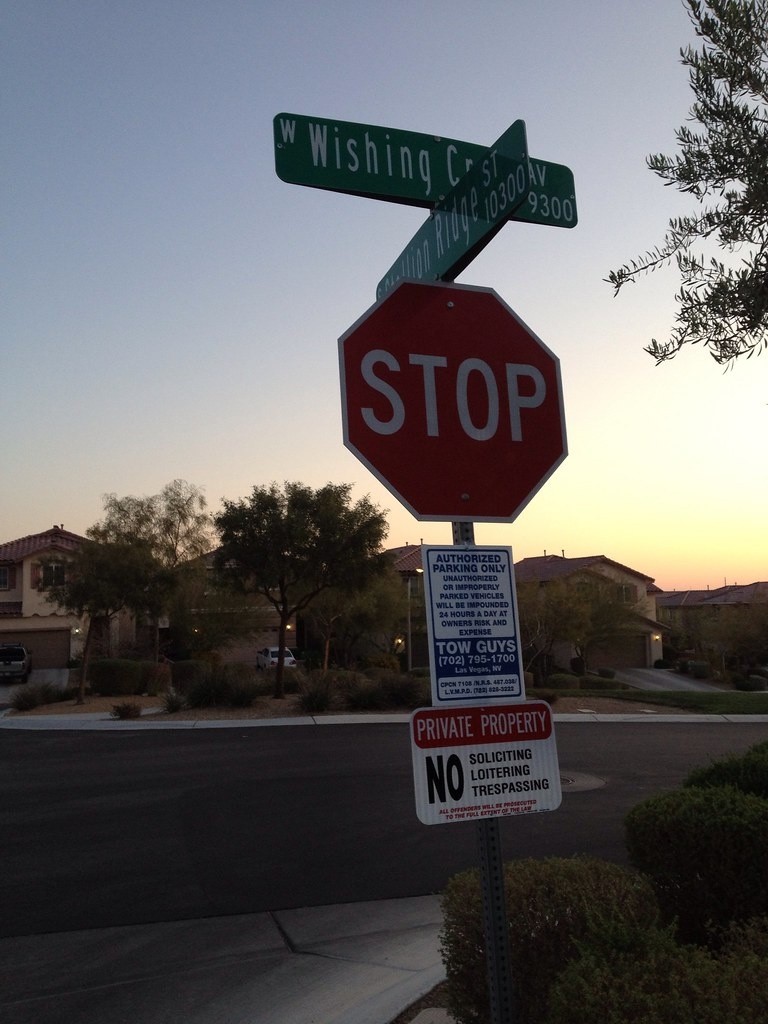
[257,646,297,673]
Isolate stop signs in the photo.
[335,277,571,524]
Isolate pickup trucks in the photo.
[0,644,35,683]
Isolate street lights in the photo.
[406,566,425,674]
